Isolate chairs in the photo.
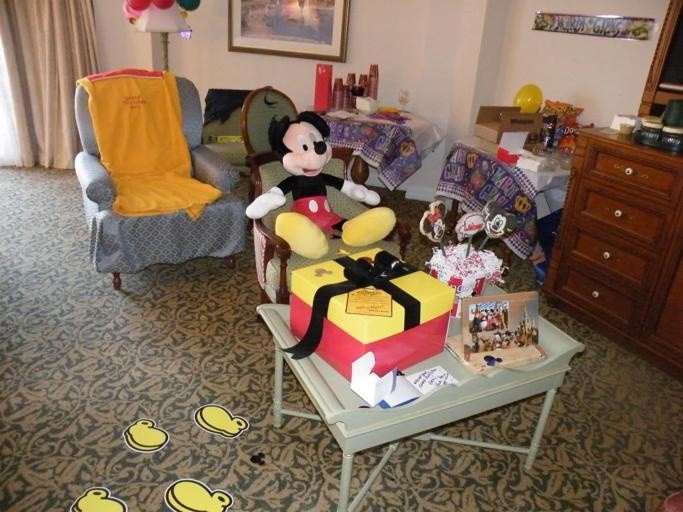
[73,64,249,290]
[236,85,403,302]
[199,89,256,177]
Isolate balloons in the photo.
[512,82,544,116]
[123,2,141,19]
[176,0,200,12]
[151,0,175,11]
[125,0,151,12]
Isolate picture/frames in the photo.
[228,0,351,63]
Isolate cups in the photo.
[619,125,635,136]
[333,65,378,110]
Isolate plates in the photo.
[513,84,543,114]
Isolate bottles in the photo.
[640,116,683,154]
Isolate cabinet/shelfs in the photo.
[540,0,682,384]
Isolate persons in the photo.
[469,303,537,355]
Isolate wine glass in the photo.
[398,91,407,116]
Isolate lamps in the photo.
[131,0,192,71]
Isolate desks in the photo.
[304,104,441,192]
[255,273,571,512]
[434,134,571,274]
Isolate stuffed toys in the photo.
[243,109,396,262]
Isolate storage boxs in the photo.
[472,104,546,154]
[284,248,456,378]
